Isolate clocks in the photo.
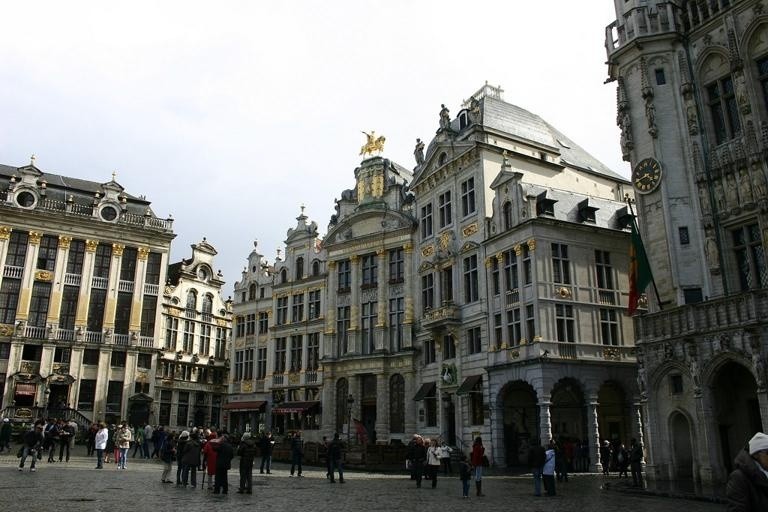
[630,154,662,196]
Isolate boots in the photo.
[475,480,486,497]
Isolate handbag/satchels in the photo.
[482,456,490,467]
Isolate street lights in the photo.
[344,393,354,449]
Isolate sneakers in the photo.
[159,479,252,495]
[17,468,37,472]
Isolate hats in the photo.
[749,431,768,455]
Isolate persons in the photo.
[361,129,376,143]
[413,138,426,165]
[439,105,451,129]
[468,96,482,123]
[725,431,767,510]
